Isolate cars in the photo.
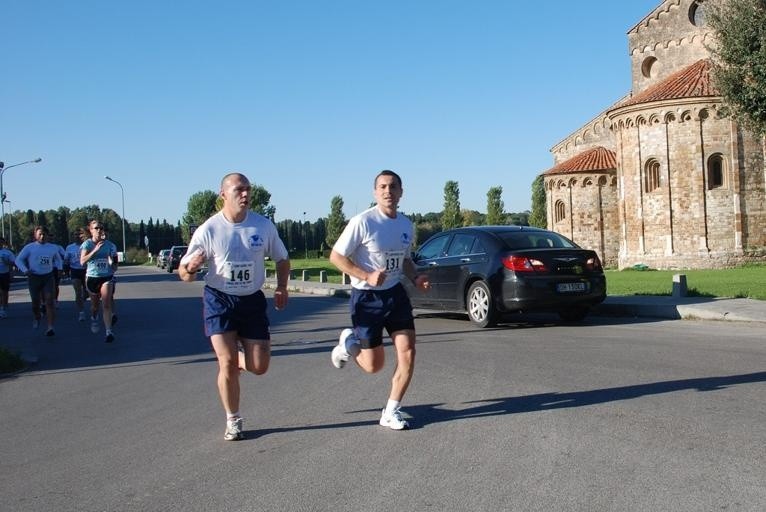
[167,245,202,273]
[156,249,170,269]
[401,226,607,328]
[108,252,126,265]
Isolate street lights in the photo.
[103,173,124,254]
[3,199,12,250]
[0,157,41,240]
[302,211,307,260]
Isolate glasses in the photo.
[91,227,104,230]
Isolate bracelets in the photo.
[184,263,194,274]
[278,284,286,288]
[411,275,418,286]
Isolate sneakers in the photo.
[332,328,357,369]
[224,417,244,440]
[33,298,60,335]
[380,407,409,429]
[79,309,117,341]
[0,305,8,320]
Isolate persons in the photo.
[178,172,291,441]
[0,219,119,343]
[329,170,432,430]
